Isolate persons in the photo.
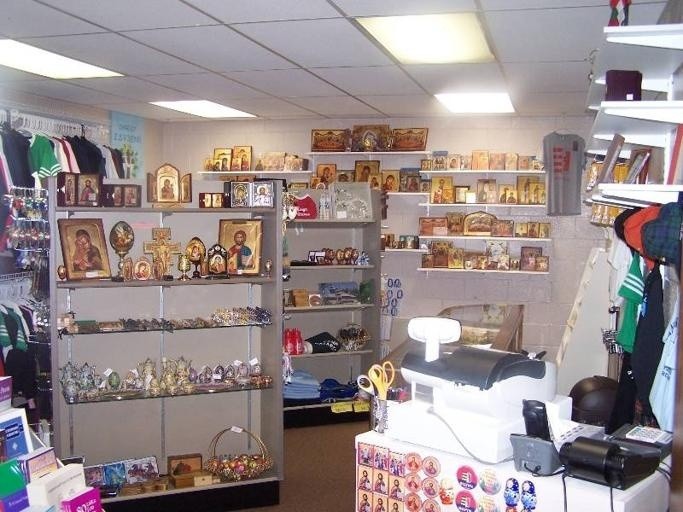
[81,180,95,201]
[383,175,394,191]
[73,229,103,271]
[358,166,371,182]
[320,167,330,184]
[227,231,254,269]
[360,448,436,512]
[161,179,174,199]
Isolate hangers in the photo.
[5,108,106,141]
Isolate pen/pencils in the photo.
[387,386,410,401]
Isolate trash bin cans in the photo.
[568,376,619,433]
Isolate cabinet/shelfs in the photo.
[42,173,281,502]
[417,168,555,276]
[575,17,682,211]
[315,145,432,257]
[284,179,385,423]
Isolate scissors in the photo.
[357,360,395,401]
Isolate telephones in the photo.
[510,399,562,477]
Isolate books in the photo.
[0,376,101,512]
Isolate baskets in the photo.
[203,427,273,482]
[337,323,370,351]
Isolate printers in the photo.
[386,315,572,465]
